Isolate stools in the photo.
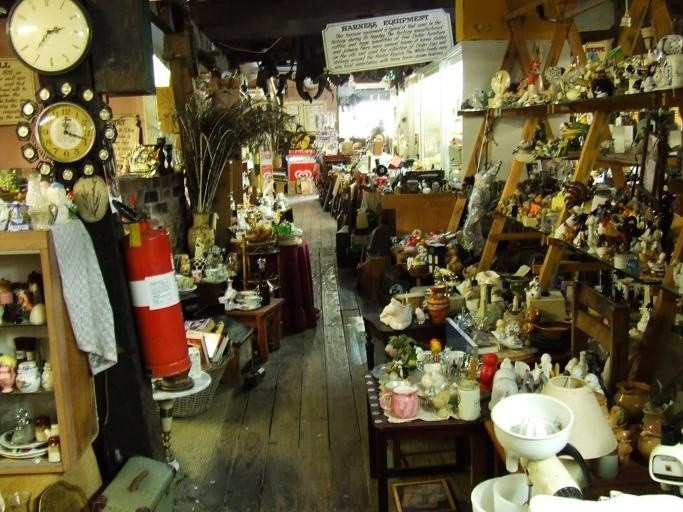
[225,297,286,361]
[361,312,486,511]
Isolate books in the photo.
[184,318,230,368]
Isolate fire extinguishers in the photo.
[109,200,191,378]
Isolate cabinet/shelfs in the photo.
[0,231,100,475]
[445,3,681,415]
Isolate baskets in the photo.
[155,345,235,419]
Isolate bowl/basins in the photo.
[490,392,574,462]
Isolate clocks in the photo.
[6,0,118,186]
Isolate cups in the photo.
[237,290,263,307]
[380,384,419,420]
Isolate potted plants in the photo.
[165,89,288,257]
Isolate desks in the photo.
[232,240,317,337]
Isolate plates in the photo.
[238,304,261,311]
[0,425,49,459]
